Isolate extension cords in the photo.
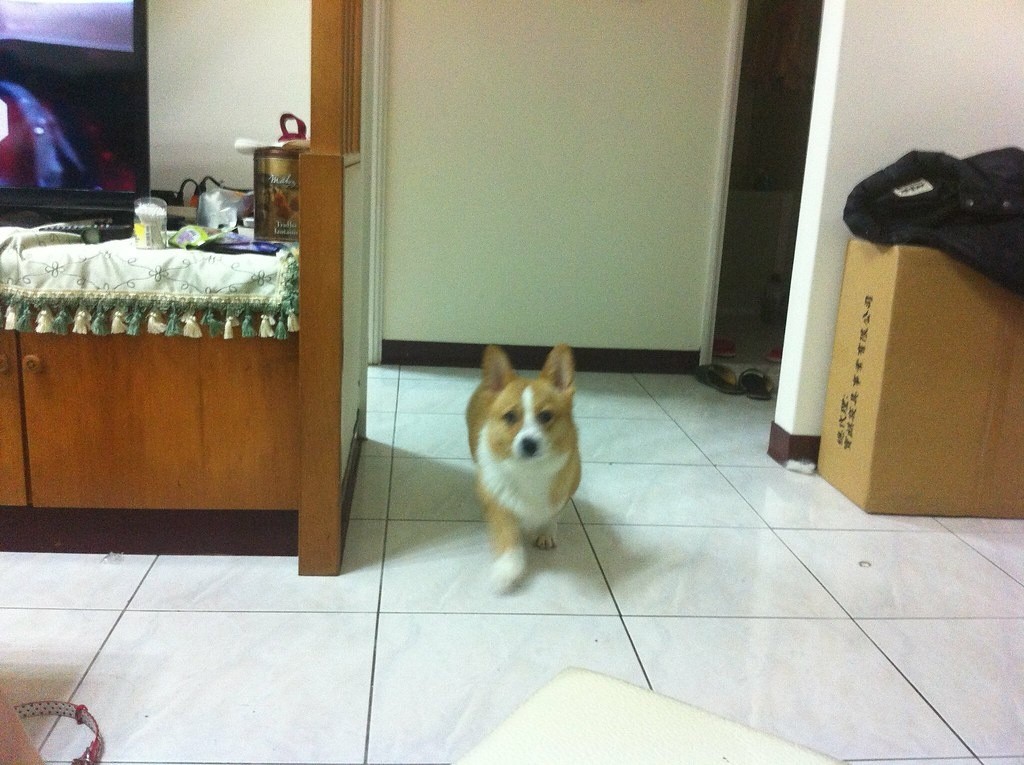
[165,205,198,222]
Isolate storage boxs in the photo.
[816,237,1024,518]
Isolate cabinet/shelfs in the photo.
[0,224,298,559]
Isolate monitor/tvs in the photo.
[0,0,151,231]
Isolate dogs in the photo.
[466,344,581,597]
